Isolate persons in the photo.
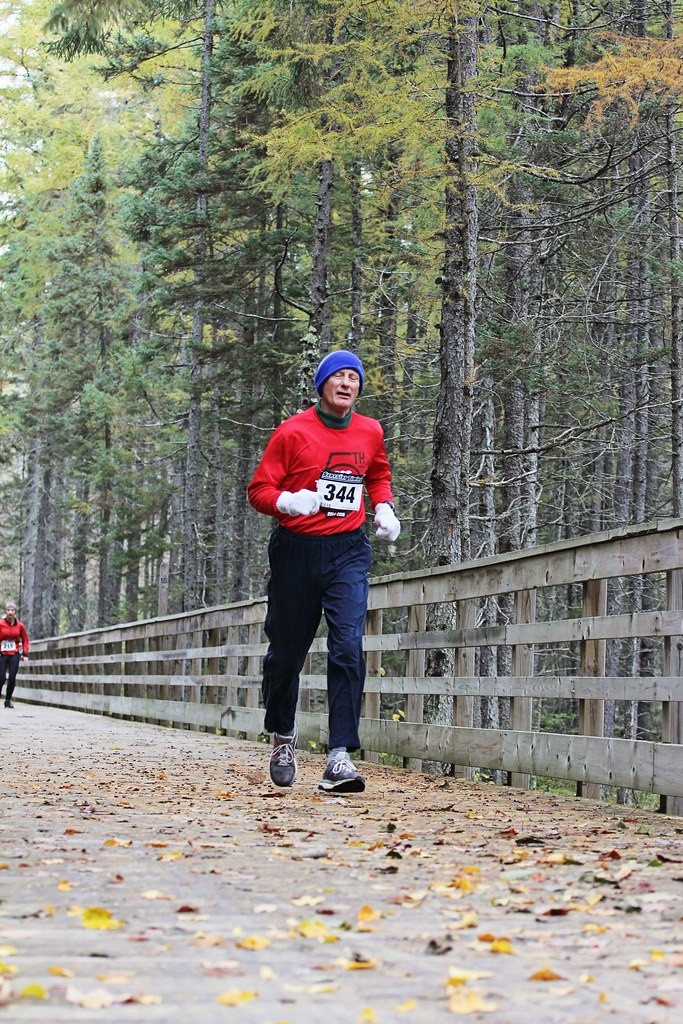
[0,602,30,708]
[248,351,401,793]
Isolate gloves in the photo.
[276,489,323,516]
[374,502,401,542]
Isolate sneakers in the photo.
[269,723,298,787]
[318,752,366,792]
[4,701,14,708]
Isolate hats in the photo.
[314,350,365,399]
[5,603,17,614]
